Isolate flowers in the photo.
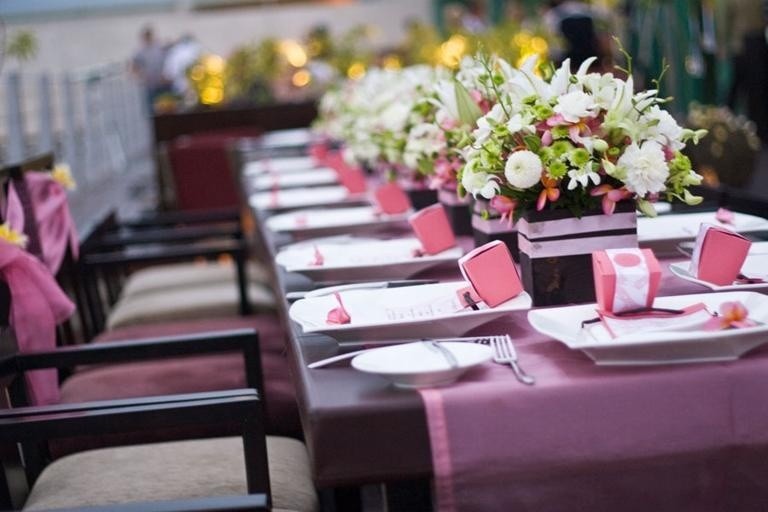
[311,39,708,228]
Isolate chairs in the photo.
[0,147,321,512]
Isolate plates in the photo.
[351,342,489,388]
[241,156,535,345]
[635,208,767,292]
[528,292,768,365]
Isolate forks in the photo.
[490,334,535,385]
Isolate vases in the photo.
[405,188,638,307]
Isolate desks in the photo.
[230,126,767,511]
[153,102,319,227]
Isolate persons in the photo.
[132,26,180,156]
[162,23,210,96]
[734,0,768,146]
[456,0,625,77]
